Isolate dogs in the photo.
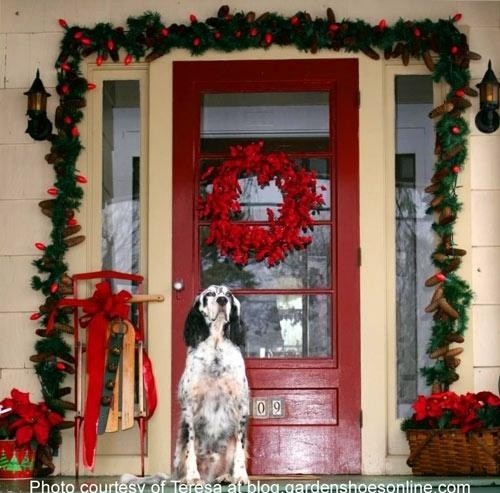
[119,284,251,488]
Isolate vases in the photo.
[0,439,38,481]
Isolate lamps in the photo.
[23,68,60,143]
[475,58,500,134]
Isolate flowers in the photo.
[399,390,500,434]
[0,388,64,447]
[200,140,326,268]
